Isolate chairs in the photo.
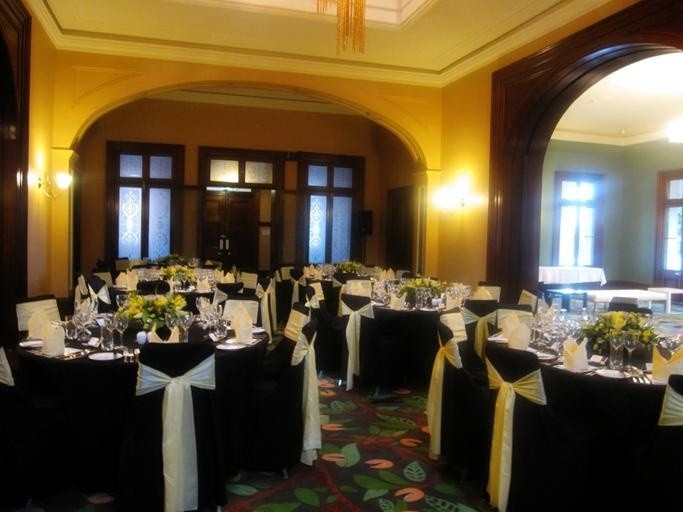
[10,256,683,511]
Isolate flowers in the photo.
[116,294,188,333]
[582,310,662,352]
[156,264,196,288]
[334,260,362,276]
[393,276,445,304]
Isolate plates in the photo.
[88,352,123,362]
[20,340,44,348]
[488,337,509,345]
[252,327,266,334]
[215,344,247,350]
[595,369,632,379]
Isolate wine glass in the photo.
[165,298,228,343]
[532,306,640,374]
[65,294,129,352]
[374,278,471,309]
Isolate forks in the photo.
[122,349,134,363]
